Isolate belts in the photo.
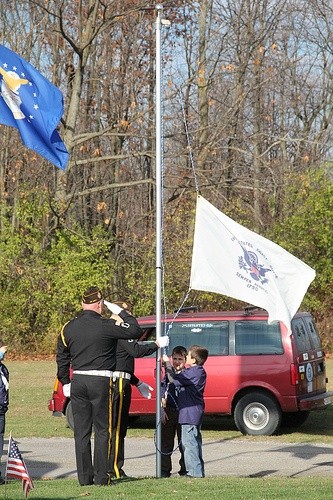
[73,370,113,378]
[113,370,132,380]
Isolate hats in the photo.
[82,286,103,304]
[114,301,132,311]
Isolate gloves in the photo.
[62,383,71,397]
[138,381,154,399]
[157,335,170,348]
[104,300,123,314]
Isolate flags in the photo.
[0,44,68,171]
[5,436,34,498]
[189,195,317,335]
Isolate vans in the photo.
[46,302,329,436]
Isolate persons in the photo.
[161,345,209,479]
[109,301,169,482]
[0,341,9,485]
[55,286,143,486]
